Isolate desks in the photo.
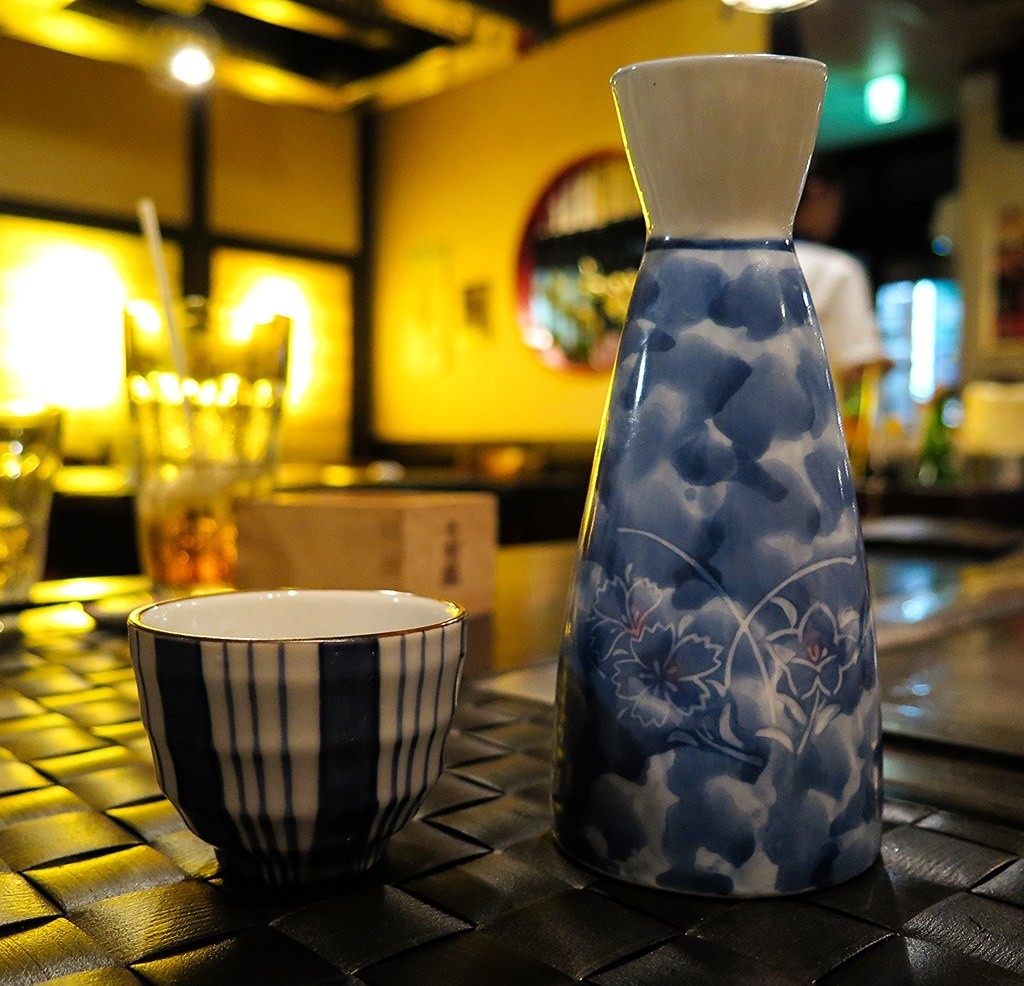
[0,573,1024,986]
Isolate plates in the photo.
[83,588,238,628]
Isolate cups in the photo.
[0,412,62,609]
[122,301,292,603]
[126,586,470,897]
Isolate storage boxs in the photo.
[234,488,497,623]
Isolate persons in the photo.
[794,167,893,389]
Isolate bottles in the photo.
[546,52,887,899]
